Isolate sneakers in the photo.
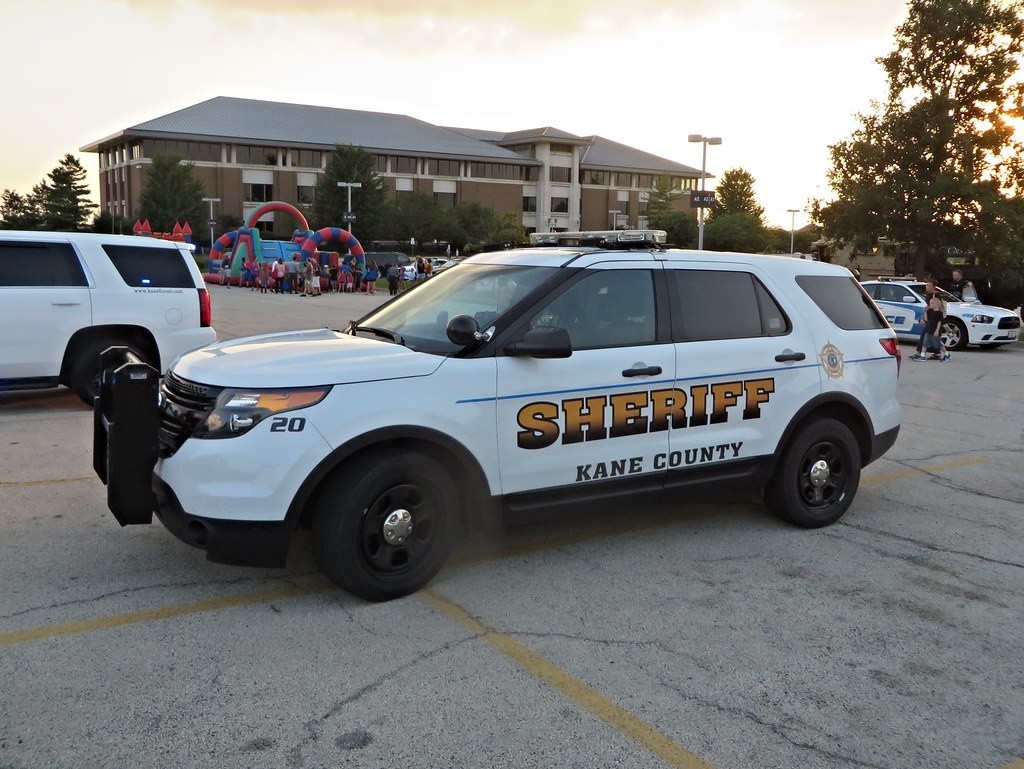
[914,356,927,362]
[940,353,952,362]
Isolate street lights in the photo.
[609,210,622,230]
[787,209,800,254]
[202,197,221,247]
[337,181,362,233]
[688,134,722,250]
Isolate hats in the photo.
[224,265,229,269]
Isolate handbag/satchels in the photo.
[933,291,947,319]
[272,264,279,279]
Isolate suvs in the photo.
[92,227,906,602]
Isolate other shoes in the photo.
[929,354,942,360]
[308,291,312,295]
[908,354,920,359]
[311,293,322,297]
[299,294,306,297]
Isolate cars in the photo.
[0,229,219,404]
[858,276,1023,352]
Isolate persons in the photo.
[909,280,945,360]
[387,263,406,295]
[414,256,432,281]
[904,271,913,278]
[445,249,452,259]
[219,256,232,289]
[913,299,952,362]
[238,256,379,297]
[950,268,973,302]
